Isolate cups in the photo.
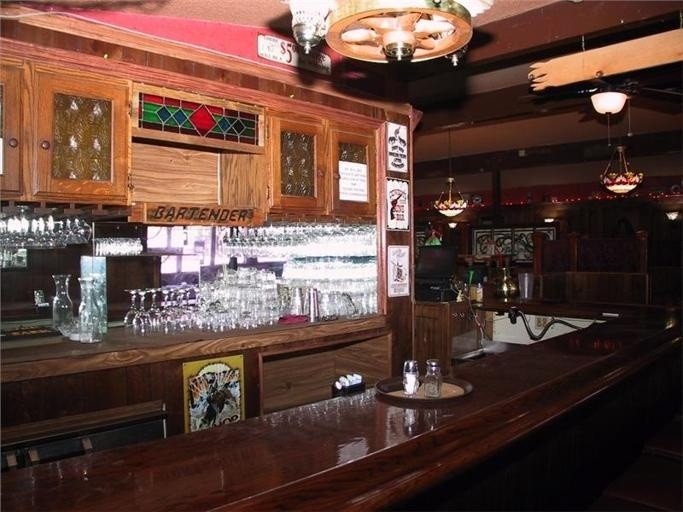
[33,290,44,306]
[518,272,535,302]
[93,237,143,256]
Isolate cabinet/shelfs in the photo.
[414,300,469,375]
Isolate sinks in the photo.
[450,342,529,364]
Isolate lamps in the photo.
[433,126,468,218]
[590,79,644,194]
[288,0,496,66]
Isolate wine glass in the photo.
[218,225,376,257]
[0,208,91,268]
[124,257,377,335]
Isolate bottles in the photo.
[497,268,520,302]
[424,359,442,397]
[465,260,483,304]
[402,360,420,394]
[52,274,108,344]
